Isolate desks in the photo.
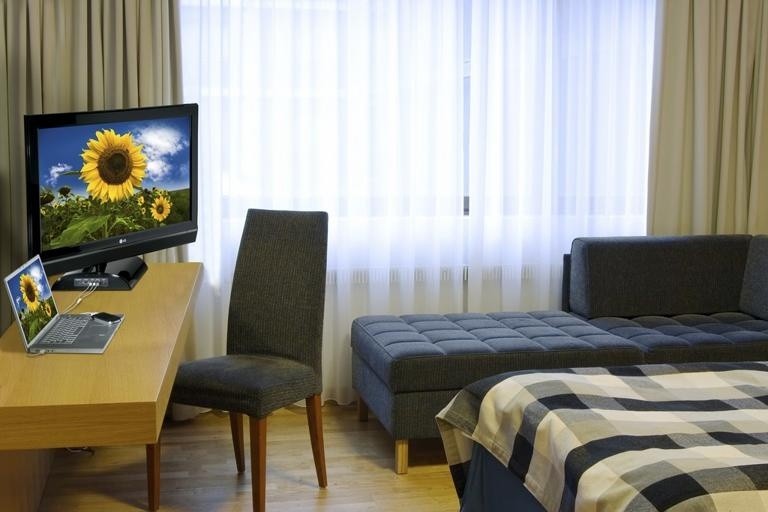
[0,260,204,512]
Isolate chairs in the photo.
[146,208,329,512]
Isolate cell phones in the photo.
[91,312,121,325]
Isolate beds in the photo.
[431,361,767,512]
[351,233,768,475]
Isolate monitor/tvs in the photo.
[3,254,125,354]
[24,103,198,291]
